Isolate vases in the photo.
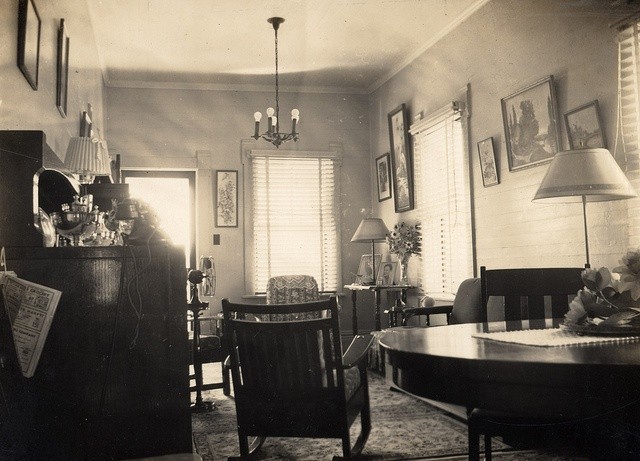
[398,252,411,285]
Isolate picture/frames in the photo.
[18,0,41,91]
[376,262,398,285]
[376,153,391,202]
[56,18,70,119]
[501,74,561,173]
[215,170,238,228]
[81,111,92,137]
[353,254,383,285]
[387,102,414,213]
[562,100,608,150]
[478,136,499,188]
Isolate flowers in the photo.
[386,220,423,256]
[563,250,640,326]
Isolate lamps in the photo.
[63,136,112,197]
[531,147,638,269]
[351,217,390,282]
[252,16,300,148]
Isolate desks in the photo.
[378,318,640,461]
[384,307,429,328]
[344,284,414,336]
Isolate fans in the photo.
[188,256,216,412]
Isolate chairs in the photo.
[262,275,322,321]
[187,314,230,404]
[221,298,375,461]
[466,264,591,460]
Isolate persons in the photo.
[379,264,393,286]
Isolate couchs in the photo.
[390,278,577,424]
